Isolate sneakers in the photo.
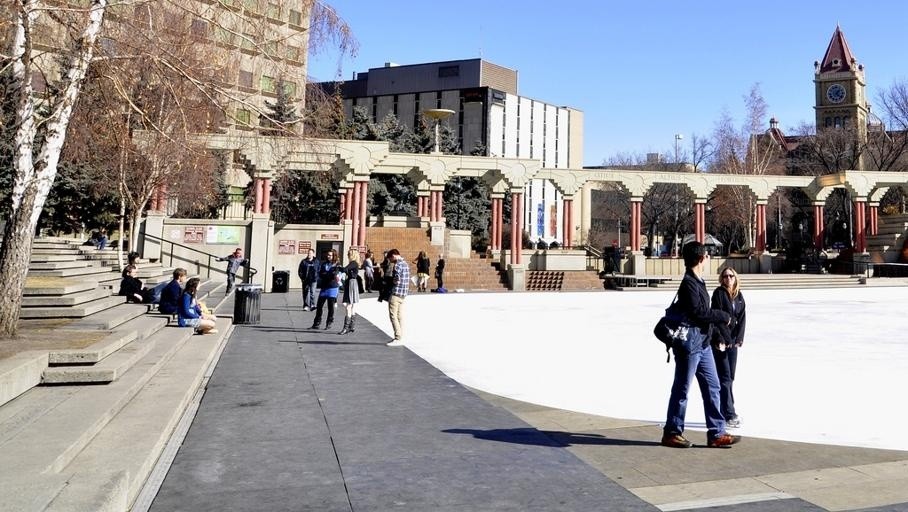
[385,339,406,347]
[726,414,744,429]
[705,433,743,449]
[302,305,309,312]
[418,287,427,292]
[661,433,695,449]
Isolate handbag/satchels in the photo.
[652,315,708,363]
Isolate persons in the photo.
[119,265,170,304]
[128,250,141,270]
[82,224,106,251]
[527,237,559,250]
[435,253,445,289]
[216,248,249,296]
[710,267,746,429]
[158,268,186,315]
[177,277,220,335]
[298,249,410,348]
[412,251,430,292]
[661,240,742,449]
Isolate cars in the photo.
[819,248,840,260]
[108,239,128,250]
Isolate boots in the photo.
[337,315,351,337]
[306,316,322,331]
[349,314,357,334]
[324,316,336,331]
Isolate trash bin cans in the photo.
[233,284,262,325]
[271,270,290,293]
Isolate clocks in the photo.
[827,84,846,103]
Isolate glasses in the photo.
[722,274,736,279]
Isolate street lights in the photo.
[674,132,683,259]
[842,222,847,248]
[773,139,784,252]
[616,186,624,249]
[799,224,805,270]
